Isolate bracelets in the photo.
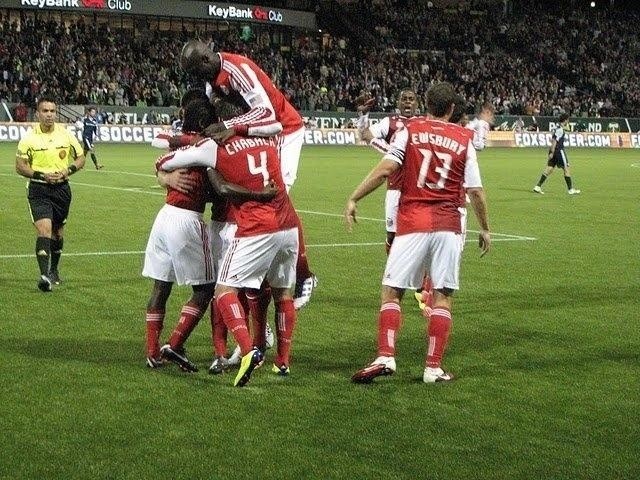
[33,171,45,181]
[549,151,553,154]
[69,164,77,173]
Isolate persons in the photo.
[357,87,437,256]
[1,1,640,133]
[81,108,104,170]
[345,82,491,383]
[532,113,580,195]
[358,95,494,316]
[181,38,318,311]
[16,98,85,291]
[143,89,319,385]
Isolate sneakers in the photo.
[534,186,544,195]
[234,350,263,387]
[423,367,454,383]
[228,343,265,369]
[38,275,52,292]
[413,290,425,310]
[293,272,318,310]
[146,357,167,368]
[96,165,104,170]
[160,342,199,372]
[209,356,231,374]
[49,270,60,284]
[265,323,275,348]
[272,362,290,375]
[351,356,397,384]
[568,188,580,194]
